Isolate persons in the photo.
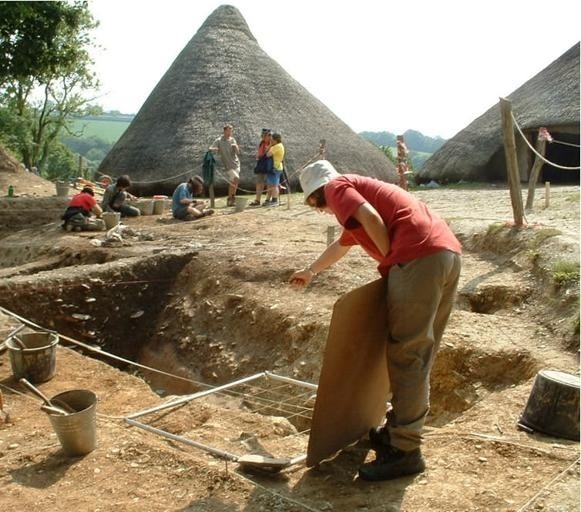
[267,134,281,206]
[250,129,272,206]
[209,124,242,207]
[171,175,215,218]
[59,188,106,233]
[286,159,463,481]
[102,176,142,221]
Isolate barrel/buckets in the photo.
[233,197,250,212]
[518,367,578,440]
[4,330,59,384]
[101,210,122,231]
[55,179,71,197]
[154,200,169,214]
[134,199,154,215]
[40,387,98,457]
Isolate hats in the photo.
[298,159,342,206]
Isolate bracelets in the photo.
[304,264,319,278]
[192,199,196,204]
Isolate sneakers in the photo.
[249,199,278,207]
[203,210,214,216]
[227,197,236,206]
[366,426,390,452]
[359,446,426,482]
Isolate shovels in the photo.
[126,418,290,468]
[0,389,10,424]
[80,235,96,239]
